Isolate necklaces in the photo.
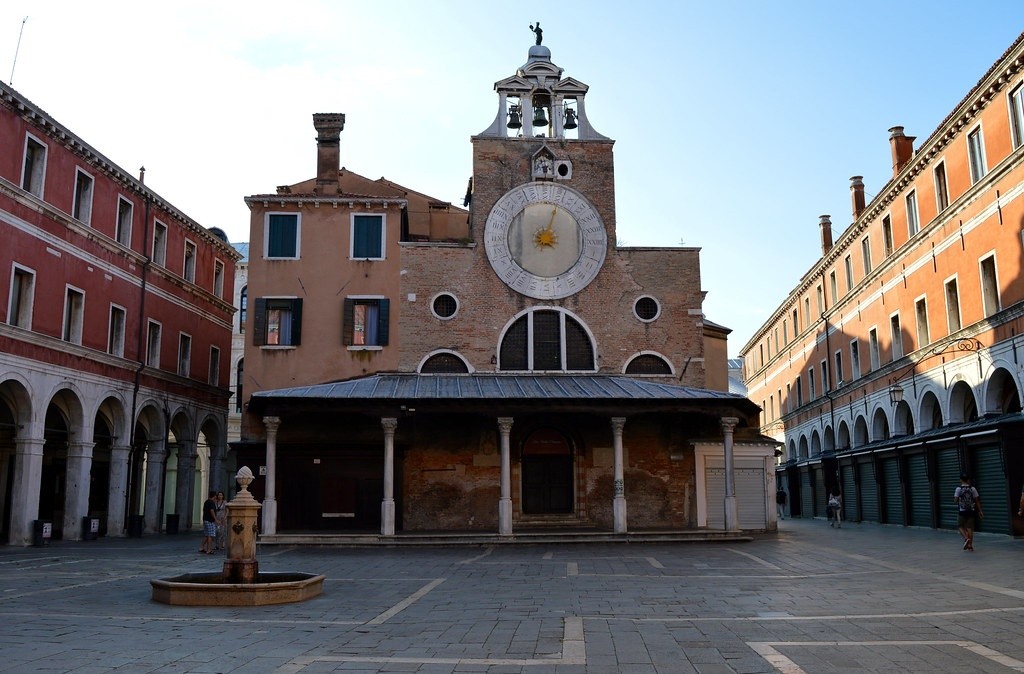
[217,500,223,512]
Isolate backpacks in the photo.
[957,486,975,509]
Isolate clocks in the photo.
[483,181,608,301]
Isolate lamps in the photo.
[889,380,904,406]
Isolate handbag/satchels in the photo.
[828,498,838,506]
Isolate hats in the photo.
[208,491,217,495]
[959,472,969,480]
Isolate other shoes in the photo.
[838,522,841,529]
[967,547,973,551]
[830,523,834,528]
[212,546,225,550]
[963,538,971,550]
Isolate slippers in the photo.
[198,549,208,553]
[207,551,217,555]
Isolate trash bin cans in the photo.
[127,516,143,538]
[32,520,54,548]
[83,516,101,541]
[166,514,180,535]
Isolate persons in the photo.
[198,491,221,555]
[776,486,788,520]
[953,472,984,552]
[828,488,843,528]
[213,491,229,552]
[1017,484,1024,528]
